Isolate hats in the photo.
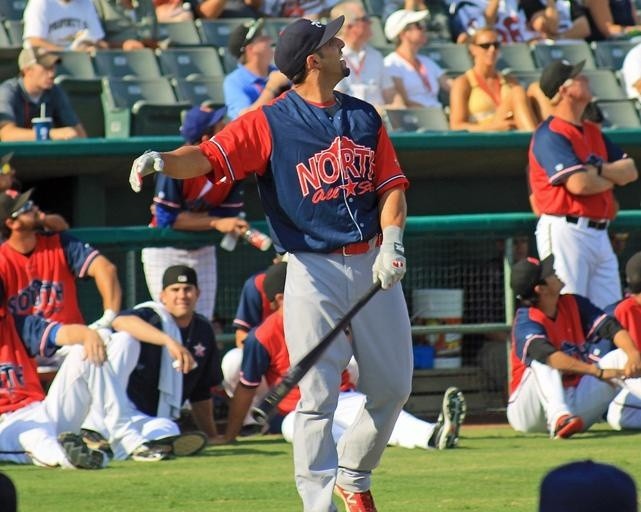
[512,255,555,301]
[539,58,586,99]
[18,44,64,70]
[163,266,199,291]
[274,16,346,78]
[226,17,264,57]
[621,252,641,294]
[0,187,37,224]
[384,9,431,42]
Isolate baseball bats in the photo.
[252,278,383,424]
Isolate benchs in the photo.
[401,361,512,425]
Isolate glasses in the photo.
[11,199,35,221]
[471,41,504,49]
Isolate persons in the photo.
[0,188,466,470]
[140,100,251,430]
[1,473,17,512]
[528,58,639,360]
[130,15,414,512]
[461,230,640,441]
[538,459,638,512]
[1,0,640,143]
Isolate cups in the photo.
[30,116,54,142]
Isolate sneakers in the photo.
[554,414,585,440]
[55,425,208,468]
[333,482,379,512]
[428,386,469,451]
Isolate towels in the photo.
[130,301,186,424]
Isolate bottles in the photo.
[241,225,275,253]
[220,211,249,252]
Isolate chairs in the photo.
[0,0,640,143]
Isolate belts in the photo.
[329,233,384,255]
[568,214,607,231]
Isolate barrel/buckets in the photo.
[413,289,463,368]
[413,346,434,370]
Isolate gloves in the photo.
[128,149,165,194]
[373,226,408,290]
